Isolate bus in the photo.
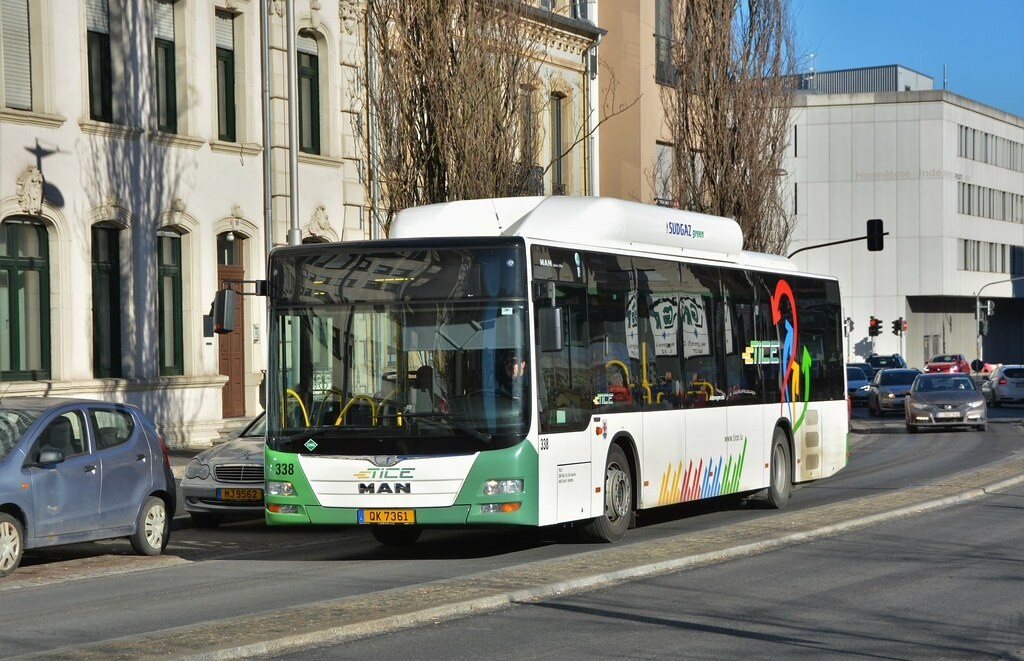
[210,193,852,551]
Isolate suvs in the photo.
[864,352,908,373]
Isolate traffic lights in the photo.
[869,319,882,336]
[892,319,899,335]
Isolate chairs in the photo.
[38,417,81,454]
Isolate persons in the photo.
[948,356,955,362]
[665,369,700,408]
[498,350,529,398]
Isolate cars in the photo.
[980,364,1024,407]
[867,367,925,418]
[0,395,178,581]
[177,407,270,528]
[903,372,987,434]
[923,353,971,375]
[846,362,876,408]
